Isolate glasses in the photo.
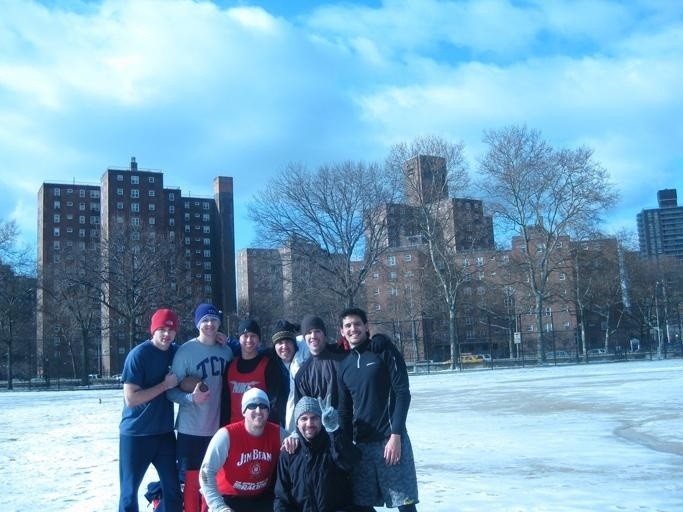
[245,403,268,410]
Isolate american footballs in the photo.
[180,375,207,393]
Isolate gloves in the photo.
[240,387,270,418]
[316,394,339,431]
[334,337,348,350]
[336,435,354,455]
[368,335,389,353]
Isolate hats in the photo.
[194,303,222,329]
[301,316,326,340]
[293,396,321,425]
[238,320,262,341]
[151,309,178,333]
[271,321,296,346]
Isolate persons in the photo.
[226,320,311,435]
[165,302,234,512]
[336,308,419,512]
[198,387,300,512]
[217,318,280,427]
[119,307,226,512]
[293,316,355,410]
[275,393,352,512]
